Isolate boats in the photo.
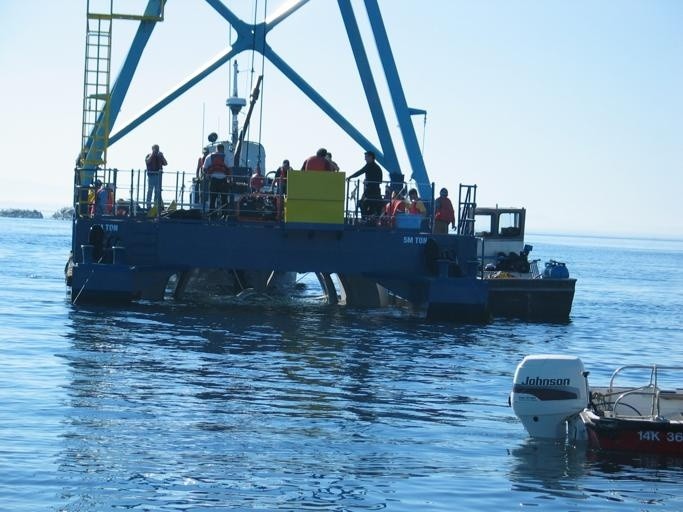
[508,355,683,455]
[460,206,577,324]
[185,59,298,298]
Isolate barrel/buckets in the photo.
[395,212,421,231]
[542,259,569,278]
